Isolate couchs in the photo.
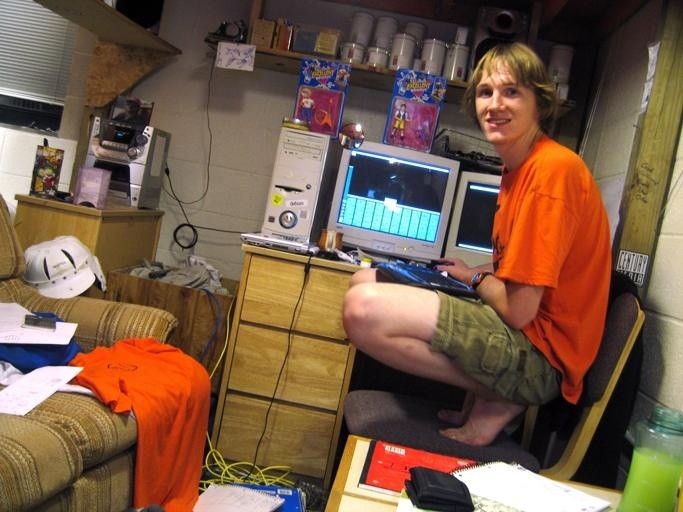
[0,190,185,511]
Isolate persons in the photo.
[343,42,614,448]
[299,87,315,129]
[390,103,412,141]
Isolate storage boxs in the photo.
[101,262,238,404]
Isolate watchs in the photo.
[471,271,494,290]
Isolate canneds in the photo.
[340,11,472,82]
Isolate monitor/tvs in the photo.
[443,171,503,269]
[326,140,460,267]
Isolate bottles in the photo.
[616,408,683,512]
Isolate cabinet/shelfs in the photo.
[239,1,572,102]
[204,245,353,492]
[13,191,163,284]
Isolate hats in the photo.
[23,235,107,299]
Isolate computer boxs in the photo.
[260,126,343,246]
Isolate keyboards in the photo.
[375,261,478,299]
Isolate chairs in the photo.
[341,281,647,483]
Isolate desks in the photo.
[321,433,681,512]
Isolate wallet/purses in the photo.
[405,467,474,511]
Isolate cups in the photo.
[318,228,344,257]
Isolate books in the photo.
[192,483,307,512]
[343,439,612,512]
